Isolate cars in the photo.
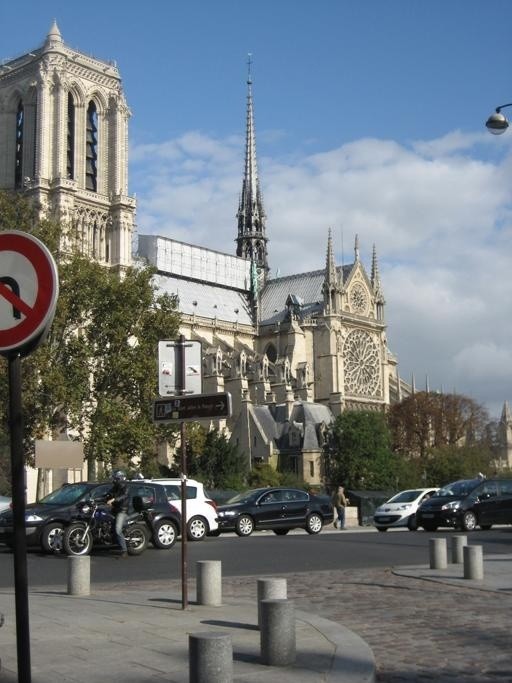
[373,487,453,533]
[0,495,13,515]
[216,487,336,535]
[0,479,177,549]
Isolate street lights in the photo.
[485,103,512,136]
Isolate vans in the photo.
[419,477,512,532]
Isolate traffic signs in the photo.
[155,392,232,421]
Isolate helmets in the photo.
[112,470,127,484]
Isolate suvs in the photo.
[132,478,219,542]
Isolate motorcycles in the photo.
[66,500,148,557]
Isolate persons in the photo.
[424,493,434,501]
[142,487,154,502]
[265,493,276,502]
[100,470,131,563]
[334,485,348,530]
